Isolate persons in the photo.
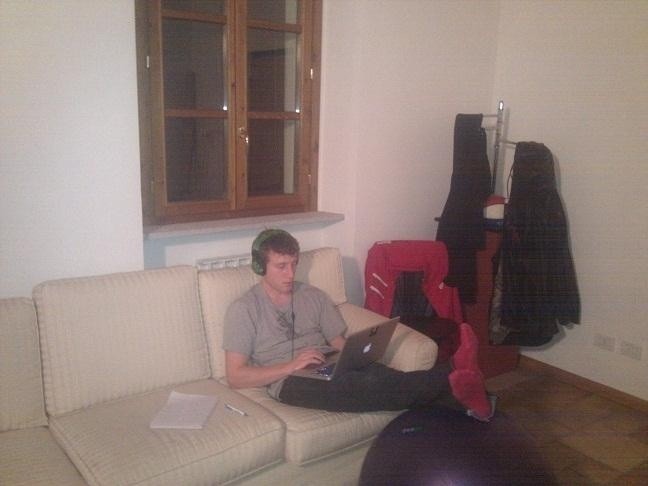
[222,228,501,423]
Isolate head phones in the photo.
[250,228,296,274]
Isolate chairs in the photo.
[364,239,480,397]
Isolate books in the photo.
[145,389,222,431]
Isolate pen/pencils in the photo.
[225,403,246,416]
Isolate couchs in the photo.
[0,248,443,486]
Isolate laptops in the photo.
[292,315,402,383]
